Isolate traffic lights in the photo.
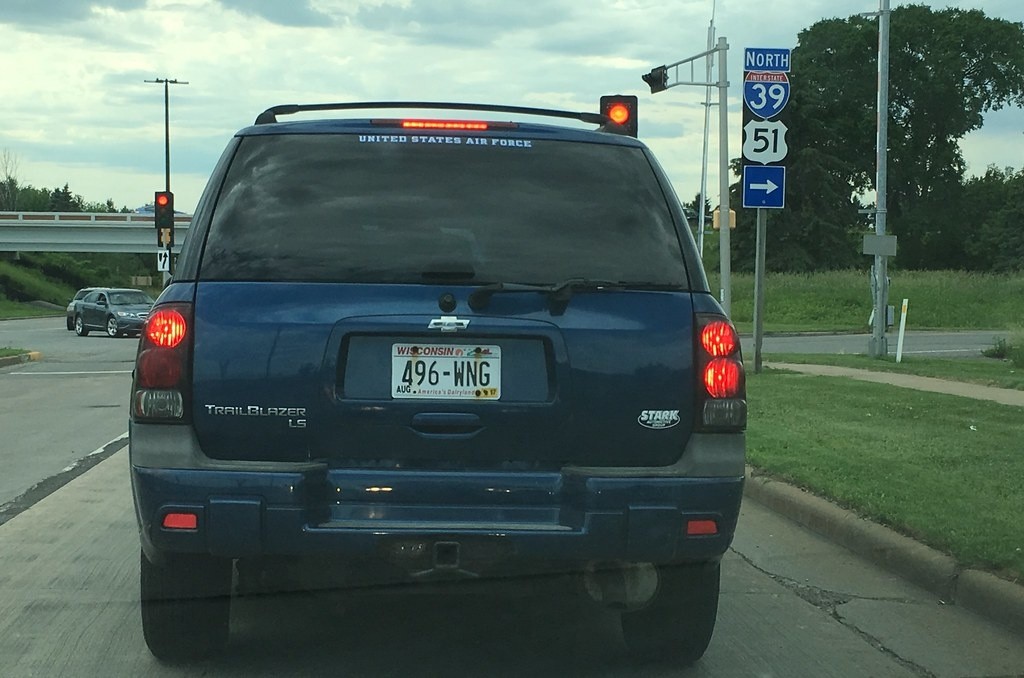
[643,64,666,95]
[154,191,175,230]
[599,95,639,137]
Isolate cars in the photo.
[66,288,111,331]
[74,290,157,338]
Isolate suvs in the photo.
[125,100,750,669]
